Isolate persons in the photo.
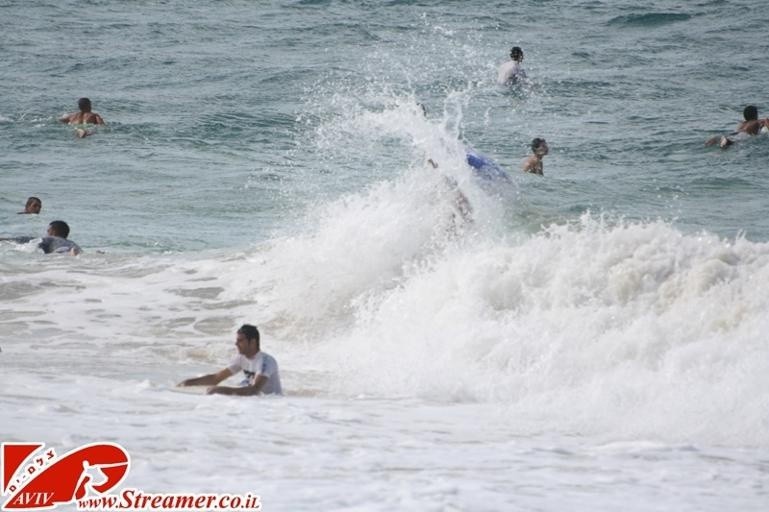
[24,196,41,214]
[704,106,769,149]
[176,323,282,396]
[64,97,103,138]
[496,46,526,86]
[522,137,548,175]
[38,219,82,256]
